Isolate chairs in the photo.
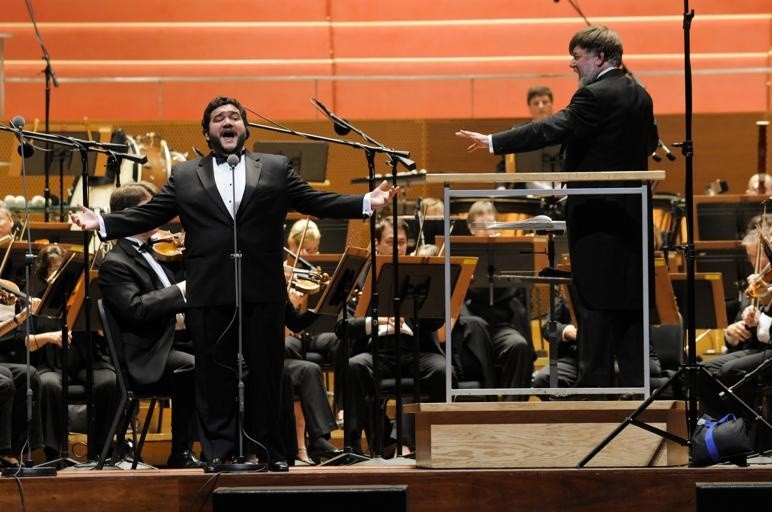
[649,321,685,398]
[753,378,772,453]
[87,298,172,469]
[315,361,335,392]
[379,375,427,455]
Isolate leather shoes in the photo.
[548,381,645,402]
[168,451,204,467]
[203,459,232,474]
[258,458,291,470]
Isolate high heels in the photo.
[306,445,349,465]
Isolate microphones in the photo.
[314,100,350,135]
[13,116,25,141]
[228,155,239,168]
[10,121,34,158]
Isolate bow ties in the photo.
[210,150,246,165]
[126,238,153,252]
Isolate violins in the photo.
[748,271,772,305]
[152,242,185,262]
[282,264,331,296]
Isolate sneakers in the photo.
[0,455,28,478]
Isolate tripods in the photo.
[314,295,372,468]
[578,3,771,469]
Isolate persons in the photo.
[1,25,772,473]
